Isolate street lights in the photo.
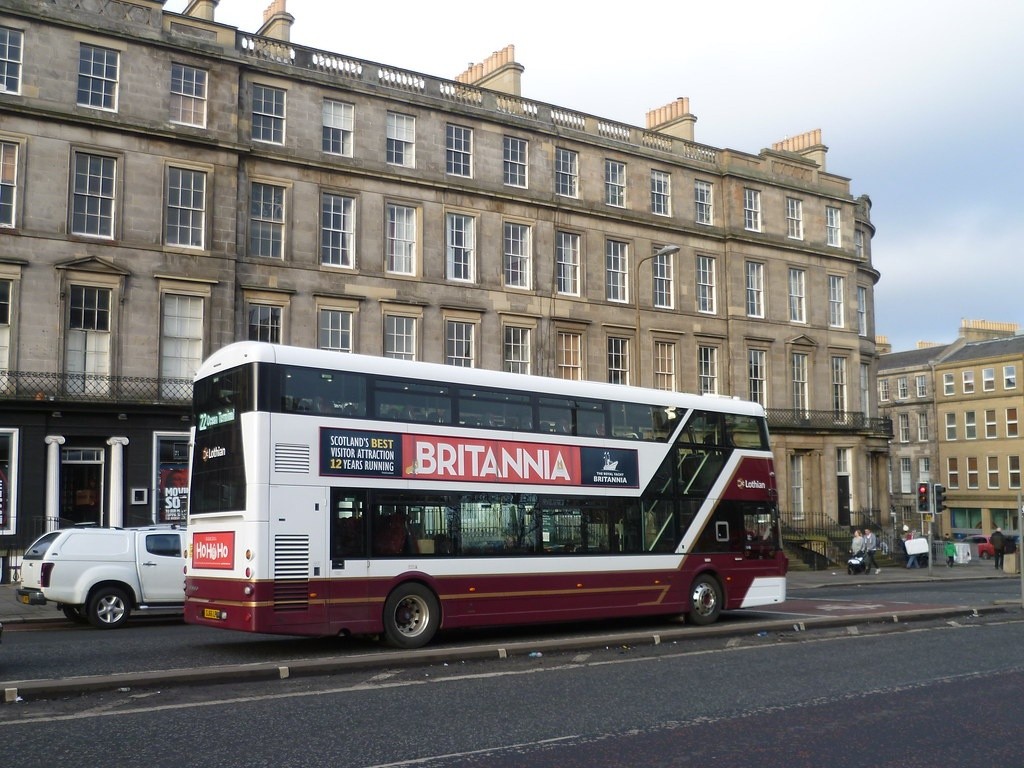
[635,244,681,387]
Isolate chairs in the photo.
[285,396,736,455]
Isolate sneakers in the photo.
[874,568,883,575]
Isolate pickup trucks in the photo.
[13,513,181,622]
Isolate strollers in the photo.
[846,550,867,575]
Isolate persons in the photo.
[862,529,881,575]
[850,530,866,557]
[905,529,919,570]
[990,527,1006,569]
[944,532,950,541]
[944,539,958,568]
[165,469,187,489]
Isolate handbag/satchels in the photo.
[416,539,435,554]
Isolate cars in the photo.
[959,534,996,561]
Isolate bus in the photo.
[180,338,793,652]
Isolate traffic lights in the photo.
[934,483,948,513]
[915,481,931,514]
